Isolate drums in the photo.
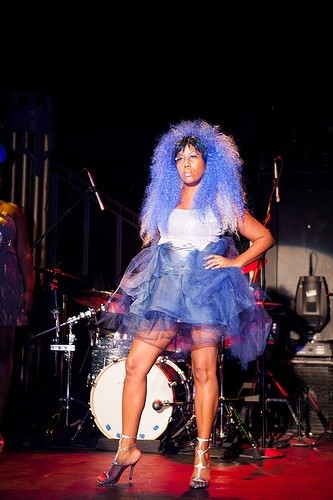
[166,328,193,351]
[88,329,136,388]
[224,331,253,351]
[88,355,191,442]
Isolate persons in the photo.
[96,117,275,489]
[0,200,34,410]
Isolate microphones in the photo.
[87,171,106,210]
[274,162,280,202]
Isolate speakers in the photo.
[294,276,330,333]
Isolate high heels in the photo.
[190,437,212,489]
[96,434,141,486]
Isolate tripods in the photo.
[165,332,301,461]
[32,307,113,445]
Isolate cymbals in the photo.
[32,266,82,282]
[254,301,283,309]
[241,258,268,273]
[71,289,129,314]
